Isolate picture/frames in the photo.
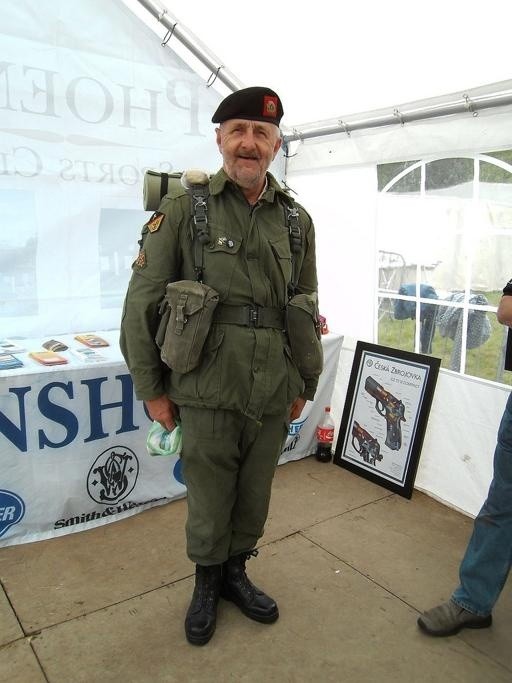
[333,337,442,500]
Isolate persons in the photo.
[416,276,512,636]
[119,87,324,646]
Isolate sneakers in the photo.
[417,599,492,637]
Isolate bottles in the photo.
[317,407,334,463]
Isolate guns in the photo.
[364,375,407,452]
[351,420,384,466]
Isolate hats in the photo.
[212,87,284,128]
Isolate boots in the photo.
[220,547,279,623]
[184,563,221,646]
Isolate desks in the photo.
[1,324,345,545]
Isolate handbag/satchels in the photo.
[155,279,221,375]
[286,293,324,380]
